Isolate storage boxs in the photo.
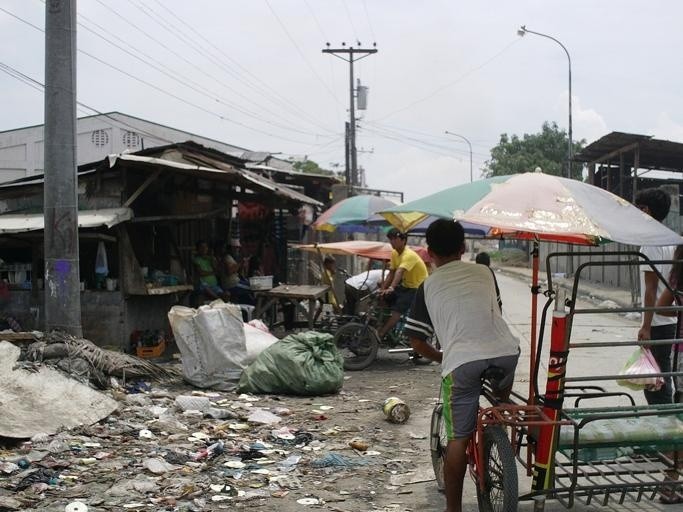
[248,275,273,291]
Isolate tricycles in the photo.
[429,247,681,511]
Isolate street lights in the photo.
[516,24,576,277]
[444,131,474,184]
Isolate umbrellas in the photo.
[311,194,398,235]
[372,166,683,477]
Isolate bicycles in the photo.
[334,286,443,371]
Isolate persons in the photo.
[191,236,265,307]
[476,252,490,268]
[655,232,683,504]
[633,188,678,405]
[401,218,521,512]
[376,228,429,344]
[321,255,343,316]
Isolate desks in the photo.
[254,285,332,331]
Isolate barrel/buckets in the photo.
[381,397,410,422]
